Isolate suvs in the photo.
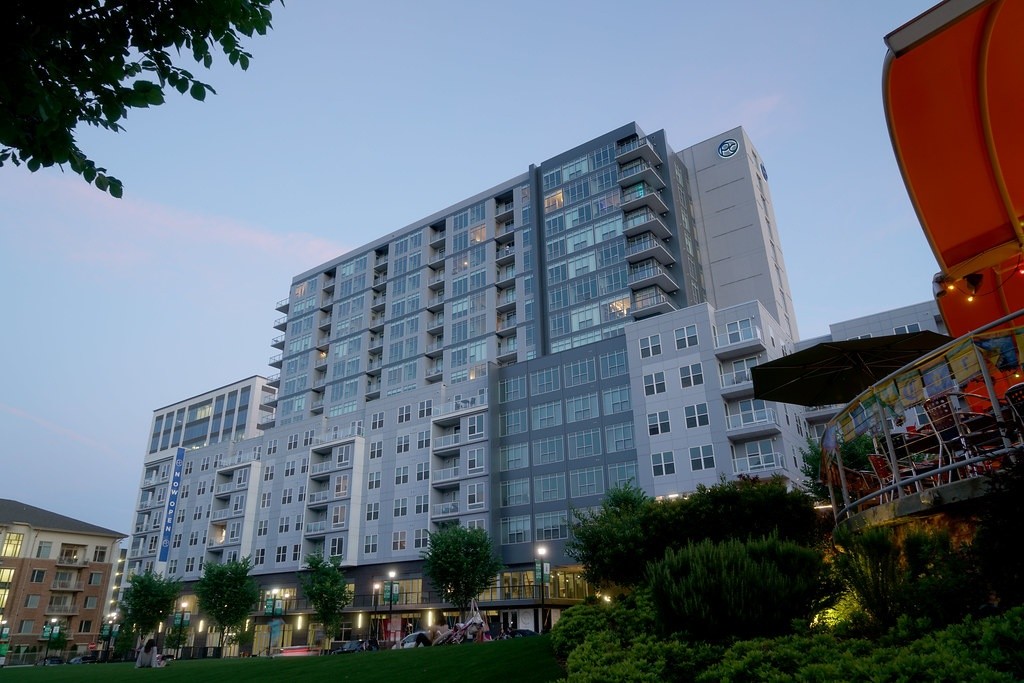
[331,637,379,655]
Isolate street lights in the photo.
[176,603,187,660]
[537,546,547,634]
[105,612,120,662]
[0,620,8,640]
[388,570,396,641]
[43,618,57,666]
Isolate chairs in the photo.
[470,398,475,407]
[854,382,1024,512]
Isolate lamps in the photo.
[964,273,985,295]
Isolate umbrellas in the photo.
[750,329,959,407]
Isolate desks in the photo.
[461,400,469,408]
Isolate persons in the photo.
[135,639,166,668]
[565,577,570,598]
[139,635,145,648]
[413,610,449,648]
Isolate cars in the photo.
[255,646,321,657]
[67,655,96,665]
[44,655,63,664]
[391,631,432,649]
[505,629,538,639]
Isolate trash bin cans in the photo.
[193,646,208,658]
[91,649,100,658]
[180,647,192,659]
[101,649,113,659]
[213,647,221,657]
